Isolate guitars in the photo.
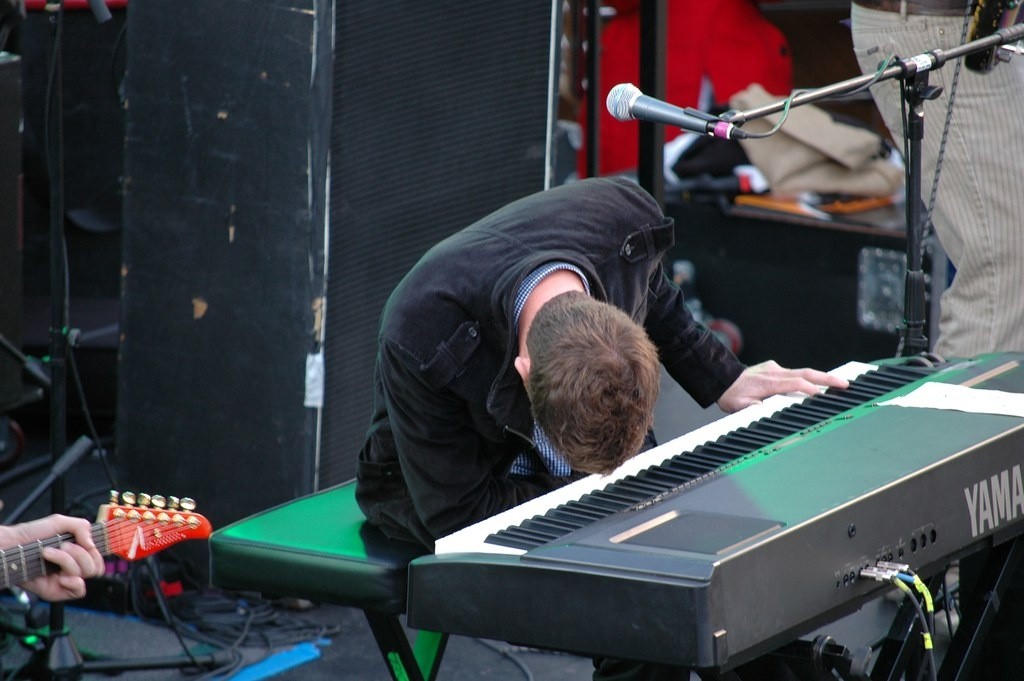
[0,489,211,595]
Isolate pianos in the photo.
[406,353,1024,672]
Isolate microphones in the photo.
[605,82,748,143]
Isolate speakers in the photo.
[120,0,558,567]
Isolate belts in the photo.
[853,0,980,17]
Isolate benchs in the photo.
[208,477,449,681]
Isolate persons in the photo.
[356,177,850,681]
[850,1,1023,361]
[0,512,104,601]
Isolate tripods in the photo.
[0,0,233,681]
